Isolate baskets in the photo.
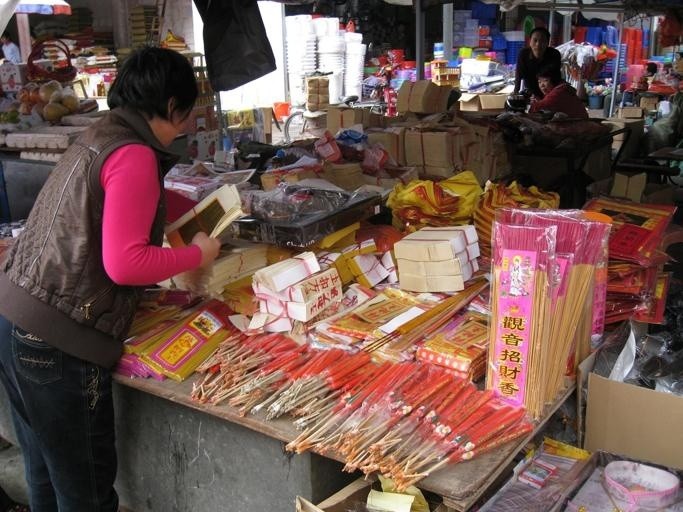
[27,38,77,81]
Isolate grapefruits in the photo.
[18,79,80,121]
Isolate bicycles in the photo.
[284,72,387,141]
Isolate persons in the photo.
[0,28,22,62]
[602,61,657,118]
[508,24,563,98]
[524,62,592,121]
[0,43,221,509]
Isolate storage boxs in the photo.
[574,326,683,473]
[452,7,493,48]
[585,177,679,214]
[458,91,507,115]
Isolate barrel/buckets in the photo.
[506,41,526,63]
[589,95,602,109]
